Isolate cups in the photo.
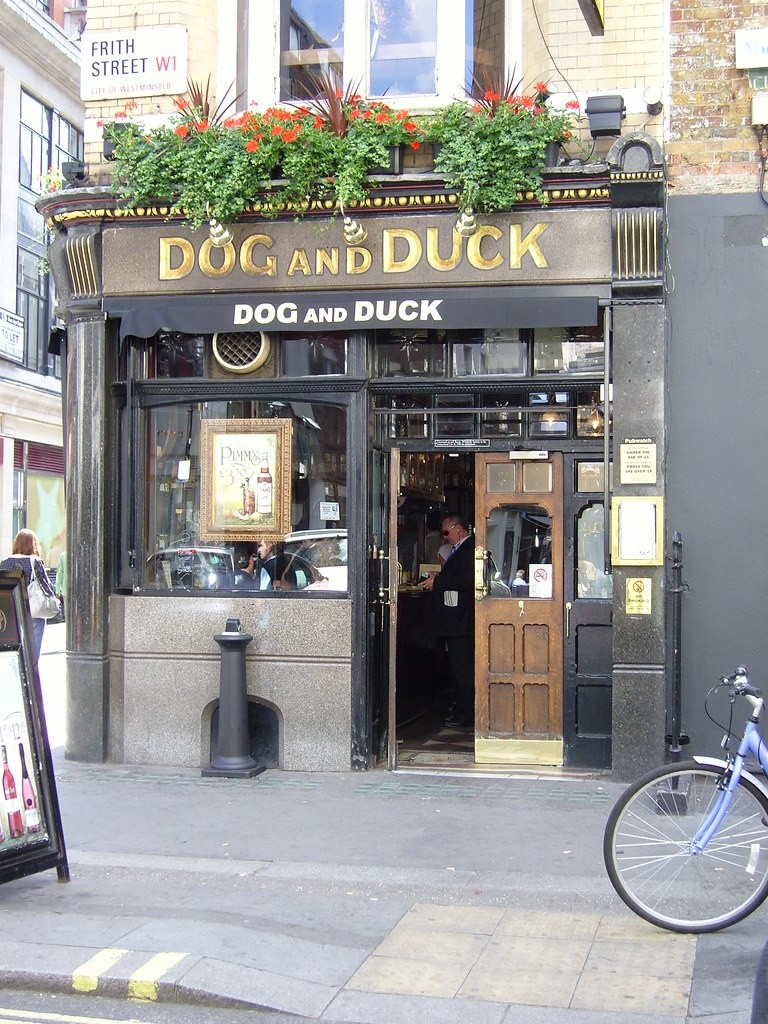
[244,488,255,515]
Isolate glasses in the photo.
[443,526,455,536]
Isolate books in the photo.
[558,350,605,374]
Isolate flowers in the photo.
[97,61,609,236]
[27,165,66,275]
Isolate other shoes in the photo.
[443,716,471,728]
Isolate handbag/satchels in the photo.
[26,556,61,620]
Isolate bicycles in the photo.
[602,666,768,936]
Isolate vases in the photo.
[254,140,561,172]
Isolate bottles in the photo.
[1,745,24,838]
[19,743,40,834]
[0,824,6,843]
[256,452,272,518]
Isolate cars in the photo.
[140,527,347,593]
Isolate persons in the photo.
[417,510,475,729]
[210,534,345,591]
[0,528,68,666]
[487,555,613,599]
